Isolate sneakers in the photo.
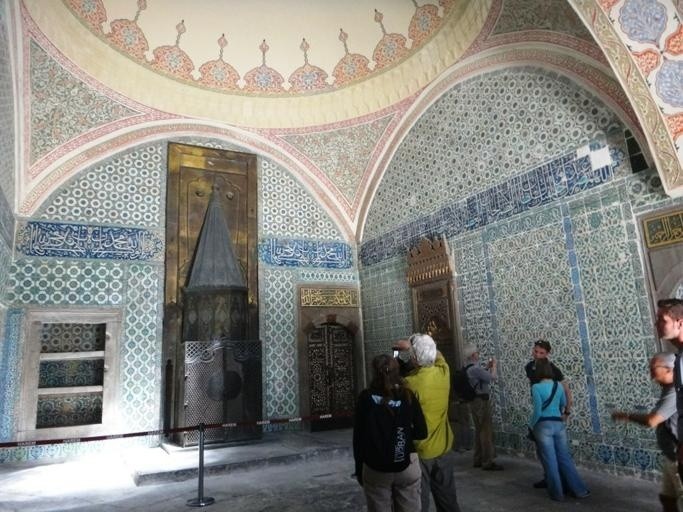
[474,462,504,472]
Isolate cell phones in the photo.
[391,348,400,358]
[488,357,493,367]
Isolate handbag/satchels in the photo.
[656,423,678,461]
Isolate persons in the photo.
[524,341,591,499]
[610,299,682,511]
[392,333,461,511]
[349,352,429,510]
[462,344,505,470]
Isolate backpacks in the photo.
[370,396,414,474]
[453,364,486,403]
